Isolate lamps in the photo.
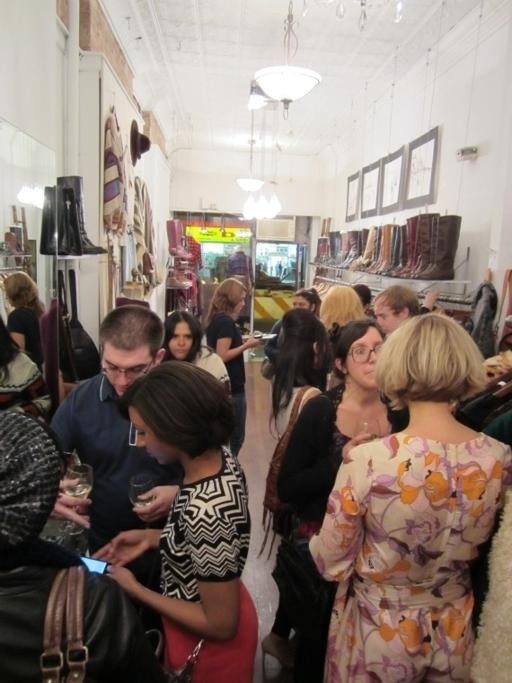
[254,0,323,119]
[247,70,269,113]
[237,108,264,193]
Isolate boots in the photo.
[343,224,407,276]
[39,176,108,255]
[393,213,462,280]
[316,231,349,268]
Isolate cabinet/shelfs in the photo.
[165,254,194,317]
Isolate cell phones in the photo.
[76,555,111,575]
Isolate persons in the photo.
[1,268,266,681]
[261,284,510,682]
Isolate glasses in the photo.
[347,345,383,364]
[102,355,153,378]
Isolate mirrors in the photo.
[0,116,57,421]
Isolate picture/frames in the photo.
[403,123,443,208]
[346,170,362,221]
[378,143,409,215]
[360,159,381,218]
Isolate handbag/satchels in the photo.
[55,321,101,384]
[123,629,204,680]
[258,429,294,560]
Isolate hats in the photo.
[131,119,150,167]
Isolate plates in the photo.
[248,328,276,341]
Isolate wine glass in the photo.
[60,463,94,536]
[351,418,380,442]
[128,472,155,530]
[248,330,260,356]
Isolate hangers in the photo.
[463,370,512,411]
[0,268,7,279]
[484,269,492,283]
